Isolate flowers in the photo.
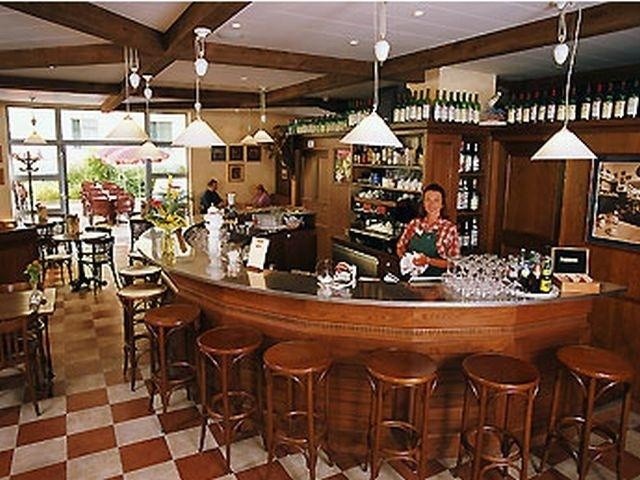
[144,176,190,249]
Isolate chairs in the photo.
[23,213,121,295]
[79,181,135,227]
[0,281,57,416]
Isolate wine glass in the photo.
[440,251,527,307]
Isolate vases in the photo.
[159,231,176,256]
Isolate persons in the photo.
[253,184,271,209]
[395,183,462,278]
[200,178,224,214]
[250,185,259,206]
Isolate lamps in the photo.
[23,97,47,145]
[529,2,598,162]
[240,84,275,146]
[101,28,228,164]
[338,2,404,148]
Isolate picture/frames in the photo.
[583,153,640,253]
[211,145,261,183]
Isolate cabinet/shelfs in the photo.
[331,121,493,278]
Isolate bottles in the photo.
[564,274,586,283]
[507,79,639,124]
[513,247,553,297]
[352,141,424,236]
[284,79,482,136]
[456,138,482,250]
